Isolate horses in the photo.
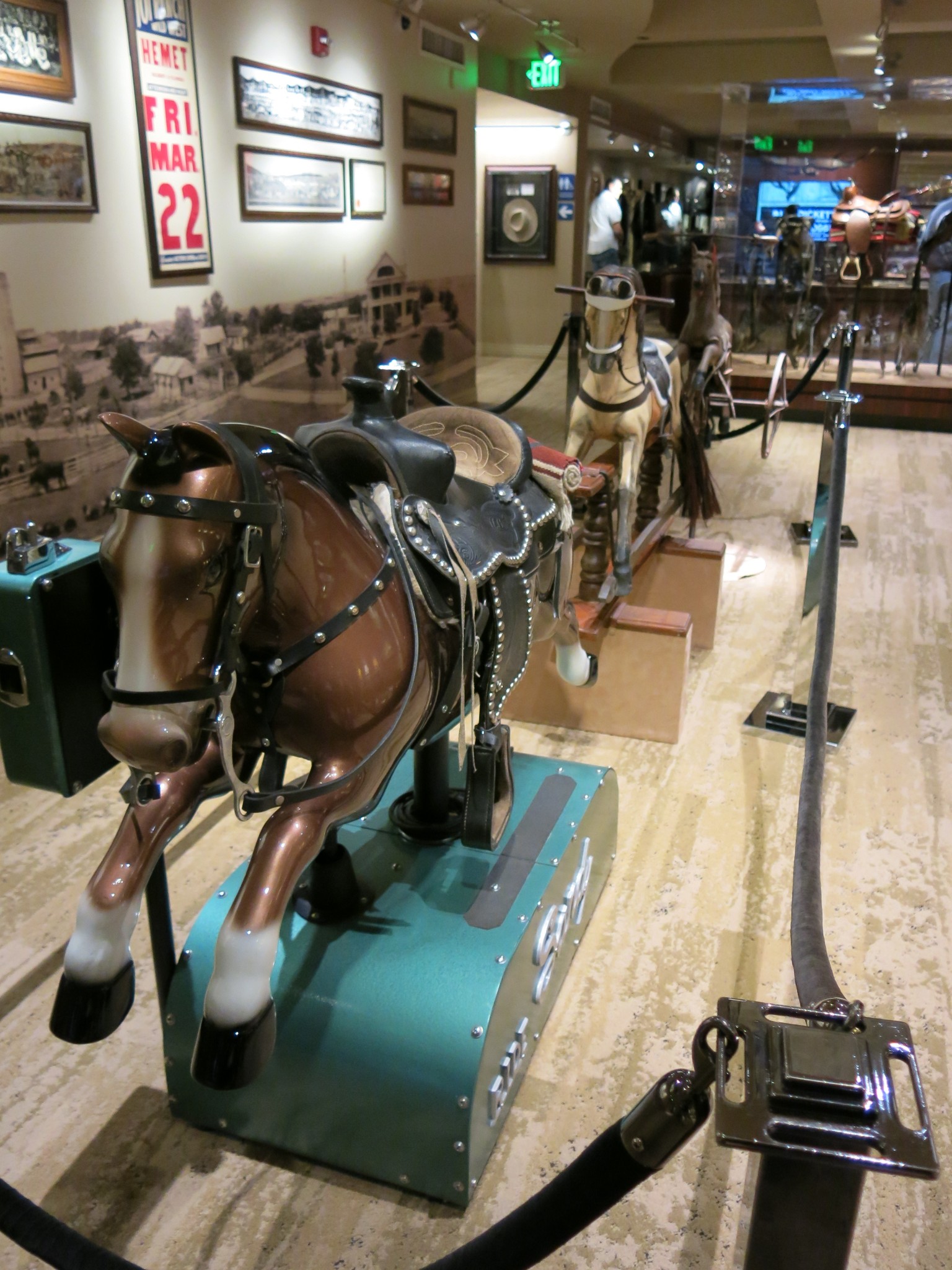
[535,238,733,602]
[48,405,599,1087]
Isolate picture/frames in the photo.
[350,159,386,220]
[238,143,346,222]
[403,96,459,156]
[124,0,214,279]
[401,164,455,208]
[233,56,384,147]
[0,112,99,211]
[0,0,77,101]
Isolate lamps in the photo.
[535,41,557,67]
[873,42,887,76]
[459,15,485,41]
[632,139,660,158]
[608,131,619,145]
